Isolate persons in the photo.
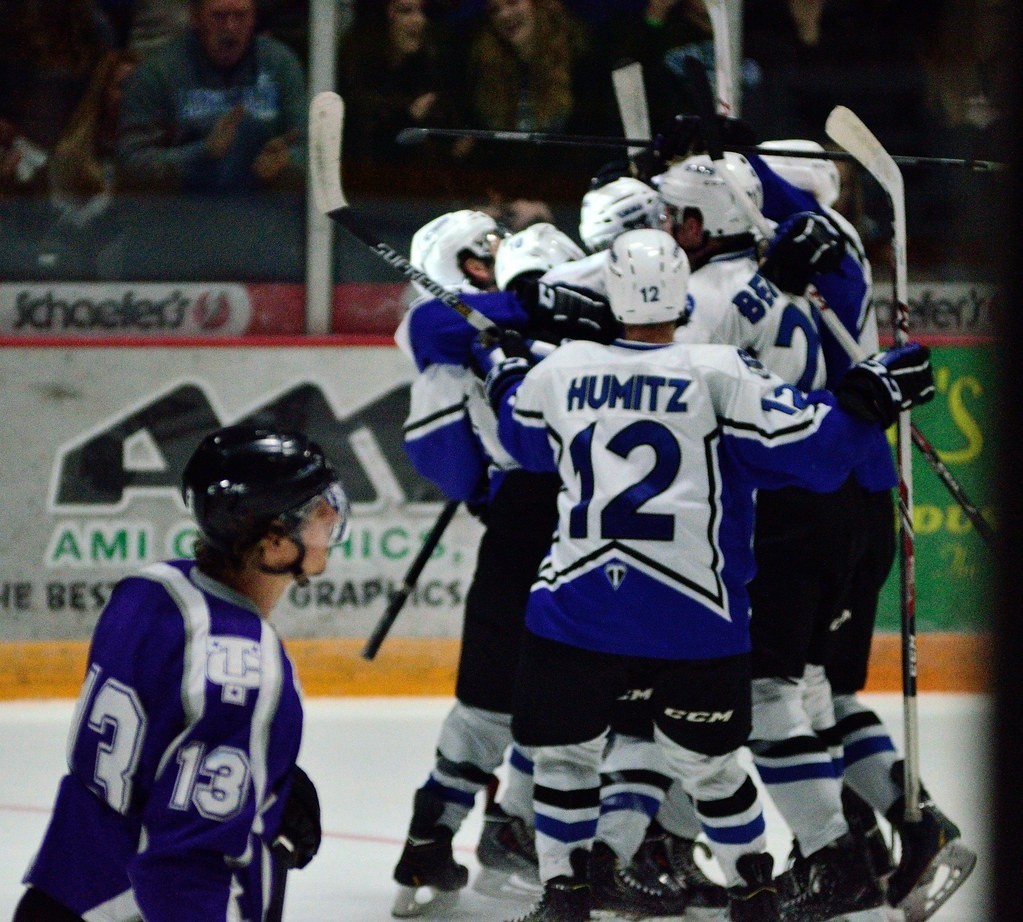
[391,111,980,922]
[12,425,346,922]
[0,0,945,247]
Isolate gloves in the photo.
[472,328,531,416]
[274,766,321,868]
[764,214,840,293]
[830,342,935,432]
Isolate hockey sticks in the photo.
[822,104,924,824]
[679,53,995,549]
[306,88,498,337]
[609,60,659,180]
[360,497,462,662]
[393,125,1004,175]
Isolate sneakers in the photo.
[589,841,646,922]
[475,772,544,902]
[500,848,593,922]
[779,833,885,922]
[658,815,729,922]
[884,760,976,922]
[391,787,468,915]
[730,853,784,922]
[616,862,684,922]
[772,824,894,907]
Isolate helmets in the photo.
[658,152,762,237]
[761,139,842,205]
[494,222,586,292]
[410,211,496,294]
[579,177,664,247]
[183,425,334,556]
[606,229,690,327]
